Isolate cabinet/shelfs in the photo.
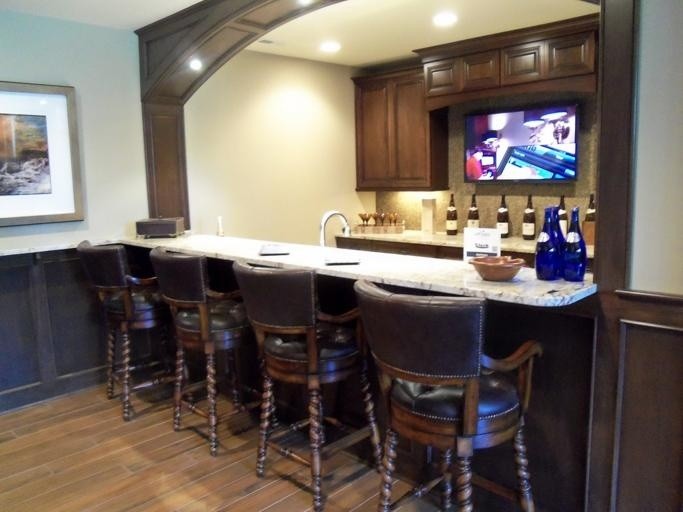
[333,232,593,273]
[408,32,500,114]
[502,9,598,96]
[348,61,451,196]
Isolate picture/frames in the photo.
[1,77,87,229]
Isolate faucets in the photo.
[319,210,350,257]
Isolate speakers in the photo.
[136,217,184,235]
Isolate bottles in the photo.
[536,204,586,282]
[584,193,596,222]
[555,194,568,237]
[468,193,480,228]
[446,193,458,235]
[496,193,510,238]
[521,193,536,240]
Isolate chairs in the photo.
[72,238,196,423]
[231,256,385,512]
[145,241,279,457]
[351,274,545,512]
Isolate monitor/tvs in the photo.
[463,101,578,185]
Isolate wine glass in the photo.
[356,212,398,226]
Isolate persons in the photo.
[466,147,494,180]
